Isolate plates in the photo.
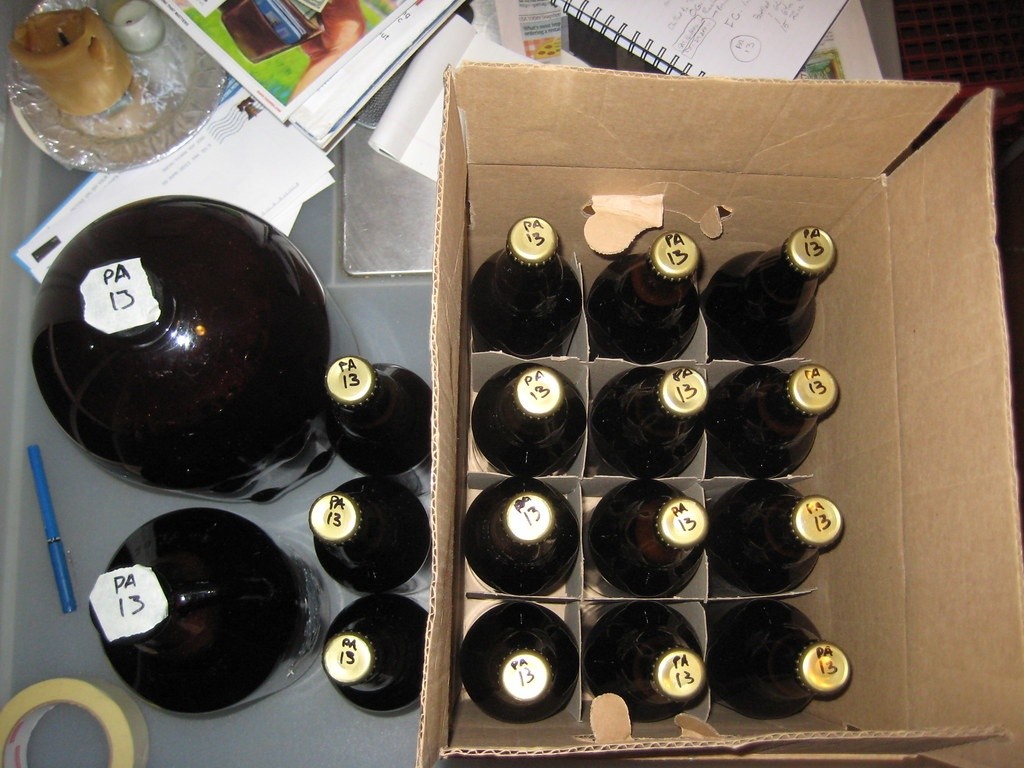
[8,0,226,174]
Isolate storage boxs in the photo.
[403,59,1024,768]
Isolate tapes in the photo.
[0,676,150,768]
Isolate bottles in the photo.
[460,600,581,723]
[323,356,432,494]
[586,230,700,365]
[704,364,839,479]
[587,364,709,479]
[471,362,587,478]
[467,216,582,359]
[29,195,359,505]
[461,477,579,597]
[581,601,704,723]
[706,598,849,720]
[581,475,710,598]
[308,474,430,599]
[706,480,844,598]
[321,594,428,712]
[90,508,328,716]
[700,226,836,365]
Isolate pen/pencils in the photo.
[28,444,77,614]
